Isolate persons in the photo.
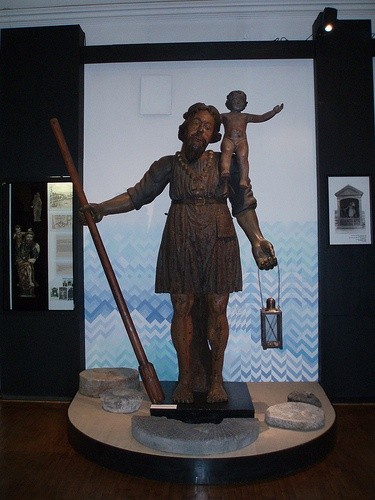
[218,90,283,190]
[13,192,42,287]
[78,102,278,403]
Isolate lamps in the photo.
[313,8,337,41]
[258,259,283,350]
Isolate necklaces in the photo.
[176,150,216,196]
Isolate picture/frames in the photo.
[327,174,373,248]
